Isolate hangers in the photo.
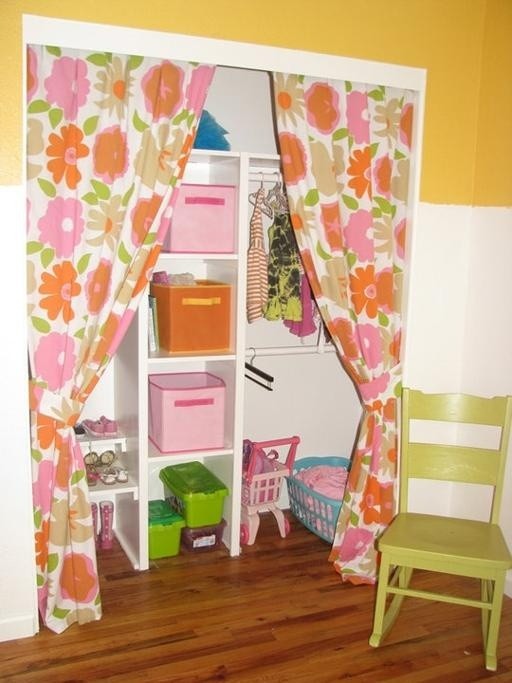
[245,346,274,392]
[249,171,289,218]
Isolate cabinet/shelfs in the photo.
[245,149,348,358]
[113,147,245,572]
[73,421,138,506]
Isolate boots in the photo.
[91,503,99,552]
[100,501,115,552]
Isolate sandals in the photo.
[84,453,99,487]
[97,450,129,485]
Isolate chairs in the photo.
[368,385,511,672]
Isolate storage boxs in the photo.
[148,500,187,559]
[148,371,227,453]
[159,461,228,526]
[149,277,232,354]
[182,517,228,551]
[161,180,239,253]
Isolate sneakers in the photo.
[100,415,117,438]
[83,420,103,439]
